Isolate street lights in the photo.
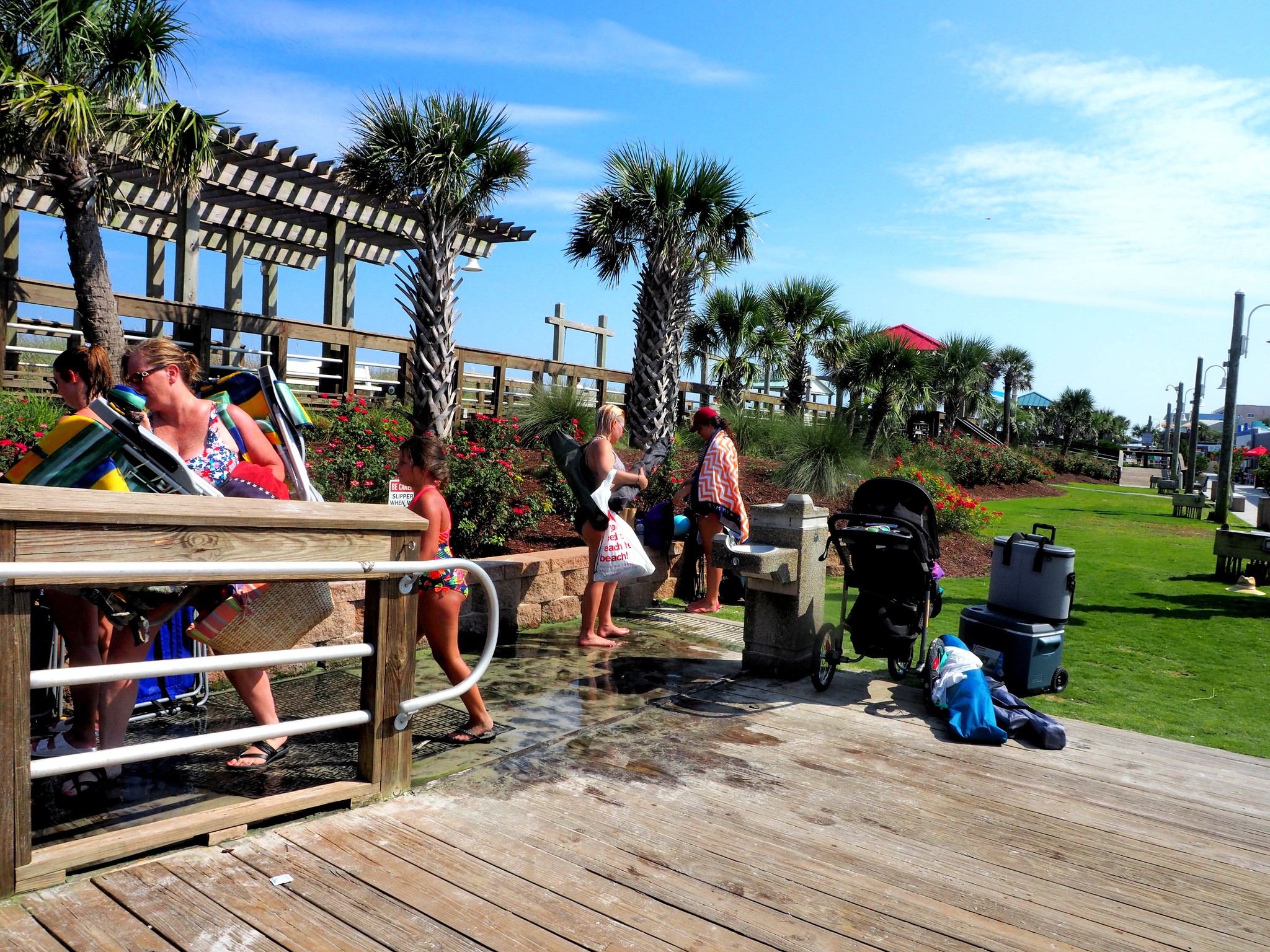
[1213,289,1270,526]
[1143,416,1161,467]
[1165,382,1206,486]
[1185,354,1228,494]
[1163,402,1185,469]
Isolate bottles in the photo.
[636,520,644,546]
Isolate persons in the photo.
[672,407,750,614]
[396,434,496,745]
[574,404,660,648]
[28,344,114,757]
[59,337,291,799]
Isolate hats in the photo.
[689,407,718,433]
[1225,575,1266,596]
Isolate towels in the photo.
[697,430,749,543]
[915,646,983,705]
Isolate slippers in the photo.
[30,731,98,755]
[225,741,289,770]
[60,767,124,799]
[47,714,99,736]
[445,725,496,743]
[684,599,723,614]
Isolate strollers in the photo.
[809,476,949,693]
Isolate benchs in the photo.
[1229,484,1247,512]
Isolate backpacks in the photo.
[640,500,675,566]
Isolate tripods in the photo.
[692,556,706,601]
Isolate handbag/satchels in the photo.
[185,579,334,675]
[986,520,1077,623]
[590,468,655,582]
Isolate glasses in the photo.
[126,364,168,386]
[615,420,629,434]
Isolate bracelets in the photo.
[635,474,640,486]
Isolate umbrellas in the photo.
[1234,445,1269,488]
